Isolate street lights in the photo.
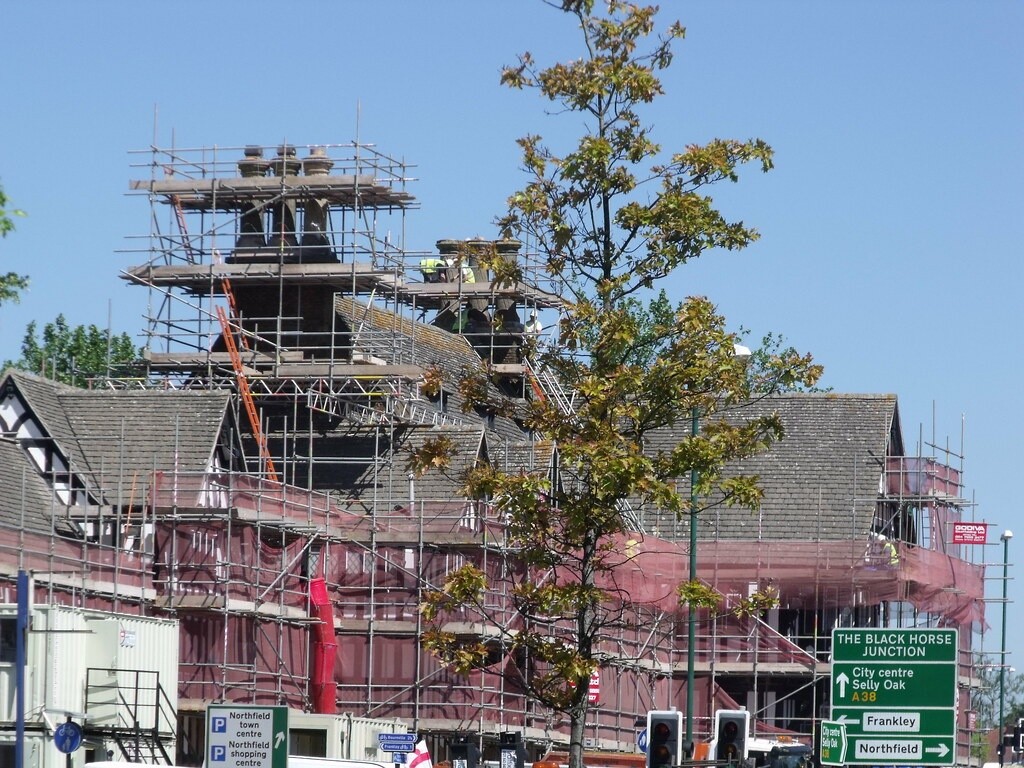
[686,345,752,768]
[999,531,1016,744]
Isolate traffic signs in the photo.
[820,719,848,767]
[392,752,409,763]
[377,733,418,744]
[379,742,415,753]
[204,704,291,768]
[829,627,959,768]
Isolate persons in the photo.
[454,256,475,283]
[419,258,454,283]
[524,310,542,363]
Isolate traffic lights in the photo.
[645,710,683,768]
[706,710,750,768]
[683,740,694,768]
